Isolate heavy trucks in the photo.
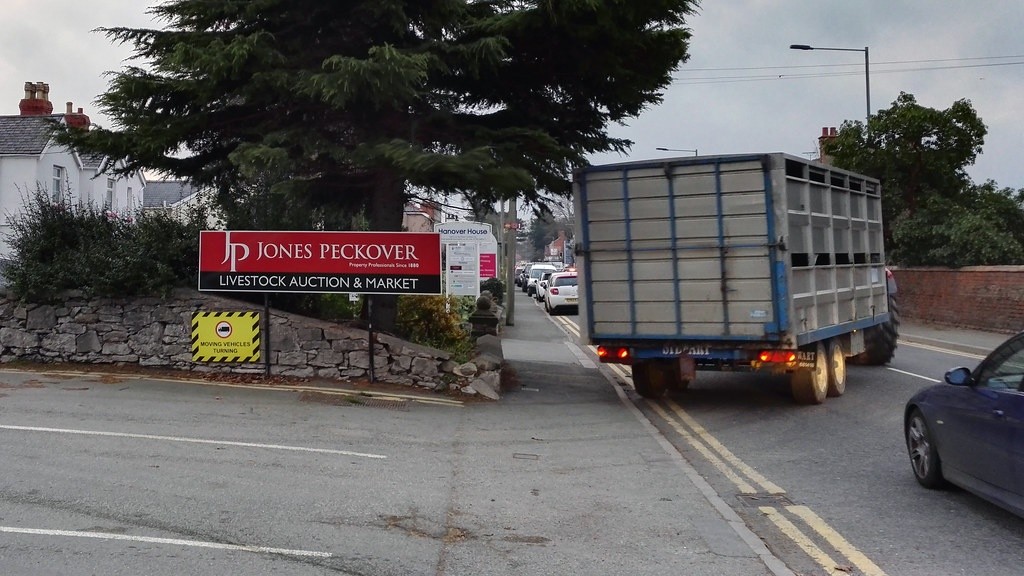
[570,150,901,407]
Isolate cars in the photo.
[534,272,552,303]
[539,271,578,316]
[902,328,1024,522]
[515,259,563,297]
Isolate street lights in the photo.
[789,43,874,177]
[656,146,697,156]
